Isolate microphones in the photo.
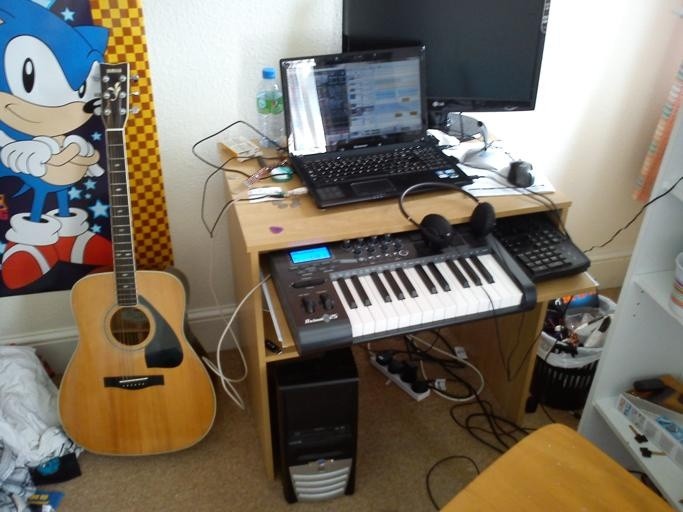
[433,419,677,512]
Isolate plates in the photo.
[574,96,683,512]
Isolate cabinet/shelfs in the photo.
[254,69,287,150]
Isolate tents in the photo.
[399,182,496,249]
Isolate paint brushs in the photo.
[369,356,431,402]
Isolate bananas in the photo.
[492,216,590,283]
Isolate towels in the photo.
[529,294,617,410]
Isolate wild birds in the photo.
[280,45,473,209]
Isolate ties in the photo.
[342,0,550,146]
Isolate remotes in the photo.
[508,159,534,187]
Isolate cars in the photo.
[669,251,683,320]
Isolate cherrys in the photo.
[629,424,648,445]
[640,447,664,458]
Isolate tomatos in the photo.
[271,222,538,358]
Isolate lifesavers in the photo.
[270,345,360,504]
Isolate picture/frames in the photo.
[219,138,606,485]
